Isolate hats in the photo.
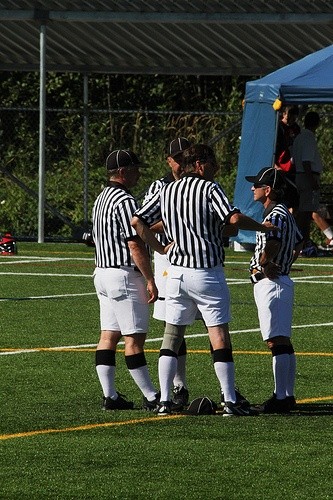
[167,136,191,158]
[106,150,148,170]
[180,397,218,415]
[245,167,285,190]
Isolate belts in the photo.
[250,273,265,283]
[110,266,140,271]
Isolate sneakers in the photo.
[143,391,183,413]
[157,400,173,415]
[250,390,299,415]
[172,386,189,404]
[221,389,249,406]
[220,401,259,417]
[103,391,134,410]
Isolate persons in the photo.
[130,143,278,416]
[246,102,333,413]
[142,137,250,407]
[92,148,161,411]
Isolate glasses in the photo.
[254,184,267,189]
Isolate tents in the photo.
[228,44,333,251]
